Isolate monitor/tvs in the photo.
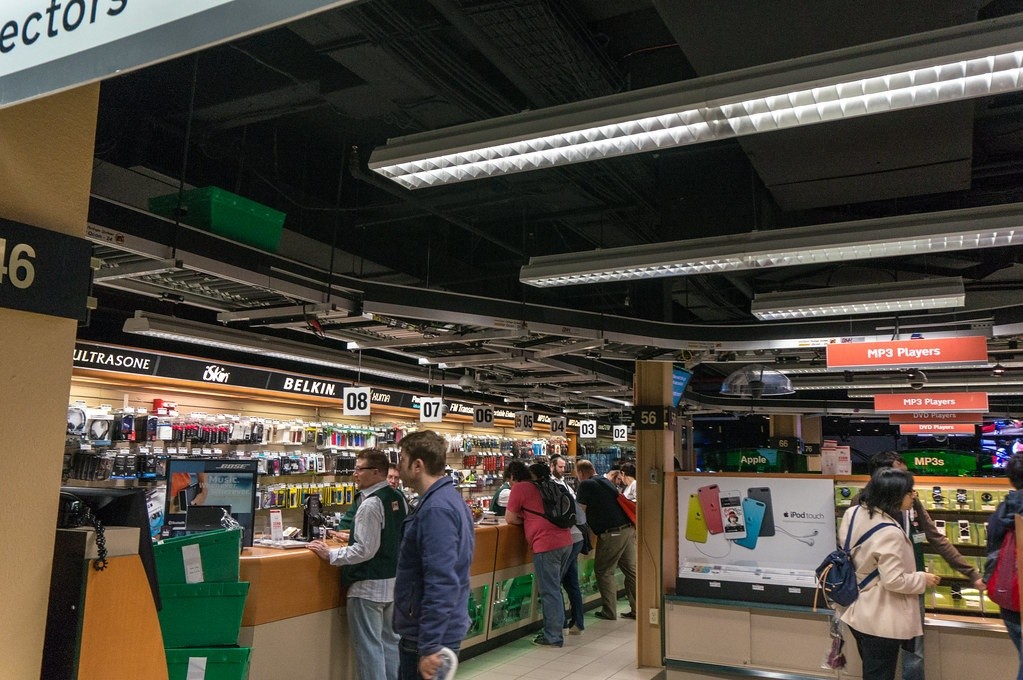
[975,419,1023,474]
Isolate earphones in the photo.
[809,530,819,536]
[799,539,814,546]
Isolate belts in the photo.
[600,524,631,533]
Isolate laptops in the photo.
[171,503,233,531]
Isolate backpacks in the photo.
[813,505,900,612]
[521,476,576,528]
[985,528,1020,611]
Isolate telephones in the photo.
[56,492,87,529]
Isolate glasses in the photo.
[354,466,381,474]
[906,490,913,497]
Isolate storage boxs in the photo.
[154,527,253,680]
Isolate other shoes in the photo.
[595,612,611,620]
[620,612,636,619]
[568,621,586,635]
[563,625,570,636]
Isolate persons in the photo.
[982,449,1023,680]
[575,458,636,620]
[304,446,415,680]
[392,430,474,680]
[489,453,586,648]
[831,448,987,680]
[333,463,416,542]
[169,473,208,513]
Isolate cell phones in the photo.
[934,520,946,537]
[952,584,961,600]
[956,489,968,502]
[685,484,775,549]
[933,487,941,501]
[958,520,970,539]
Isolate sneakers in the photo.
[530,634,562,648]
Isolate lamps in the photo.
[368,14,1023,191]
[521,204,1022,286]
[751,275,968,321]
[992,361,1007,373]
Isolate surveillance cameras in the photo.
[907,371,928,389]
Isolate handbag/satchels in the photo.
[590,476,636,524]
[577,524,593,555]
